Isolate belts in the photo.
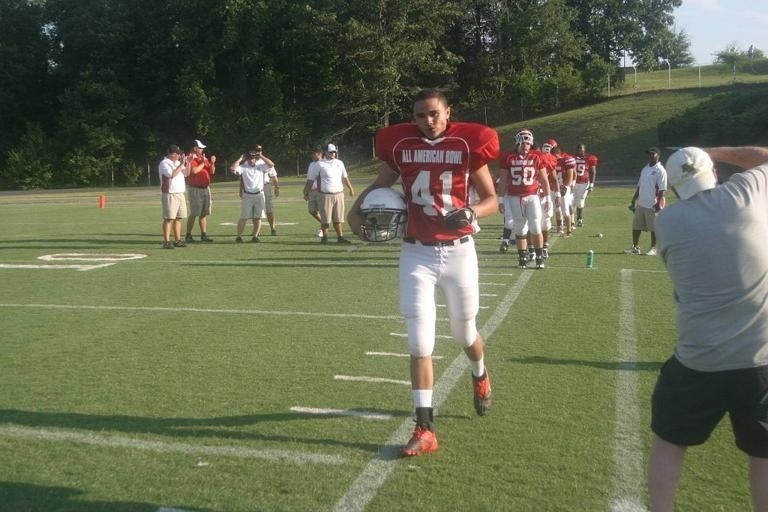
[402,236,470,247]
[244,189,262,194]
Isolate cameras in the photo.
[246,151,257,158]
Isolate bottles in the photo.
[586,249,594,268]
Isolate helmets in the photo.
[513,127,535,147]
[359,187,408,244]
[542,139,557,154]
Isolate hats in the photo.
[645,147,661,156]
[313,144,324,153]
[666,146,716,201]
[192,140,207,149]
[326,143,337,153]
[167,145,184,154]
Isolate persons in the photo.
[346,87,500,456]
[648,146,768,512]
[623,145,668,256]
[158,138,355,250]
[495,129,597,270]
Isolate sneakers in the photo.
[622,244,640,255]
[577,219,583,227]
[518,241,551,269]
[646,246,657,256]
[201,235,213,243]
[175,240,187,247]
[472,366,493,416]
[272,230,280,236]
[338,236,350,244]
[252,237,260,243]
[571,222,577,230]
[186,235,195,242]
[163,240,175,249]
[321,237,328,245]
[501,241,510,252]
[400,426,439,456]
[236,237,243,243]
[317,230,324,238]
[557,225,564,235]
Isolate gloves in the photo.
[652,203,660,213]
[627,201,636,213]
[446,206,475,229]
